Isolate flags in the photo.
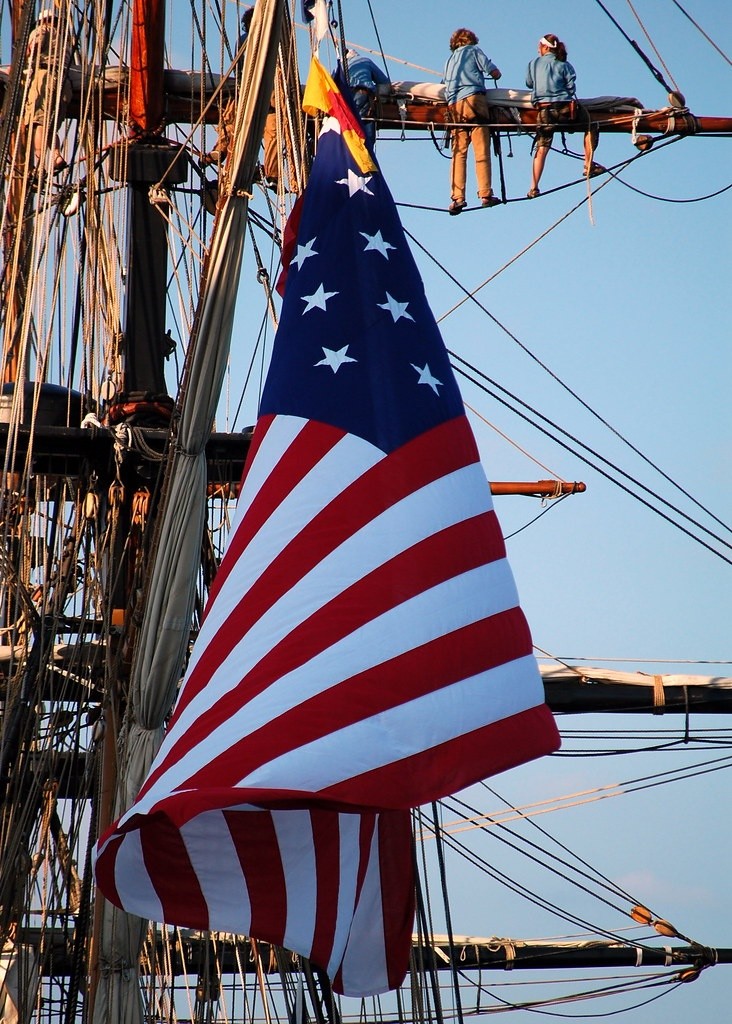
[91,54,562,998]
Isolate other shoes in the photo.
[449,200,467,215]
[527,189,539,198]
[481,196,499,207]
[583,163,604,176]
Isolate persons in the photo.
[331,49,390,152]
[525,34,606,198]
[440,28,502,216]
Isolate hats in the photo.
[346,49,360,59]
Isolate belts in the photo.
[351,88,369,95]
